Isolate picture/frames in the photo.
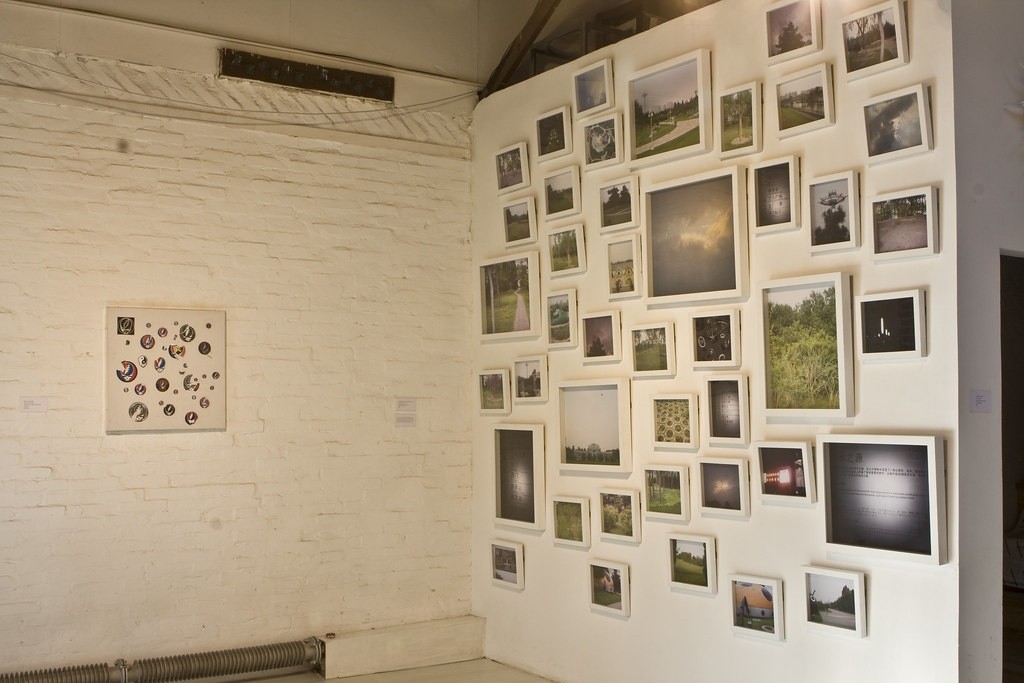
[476,1,953,643]
